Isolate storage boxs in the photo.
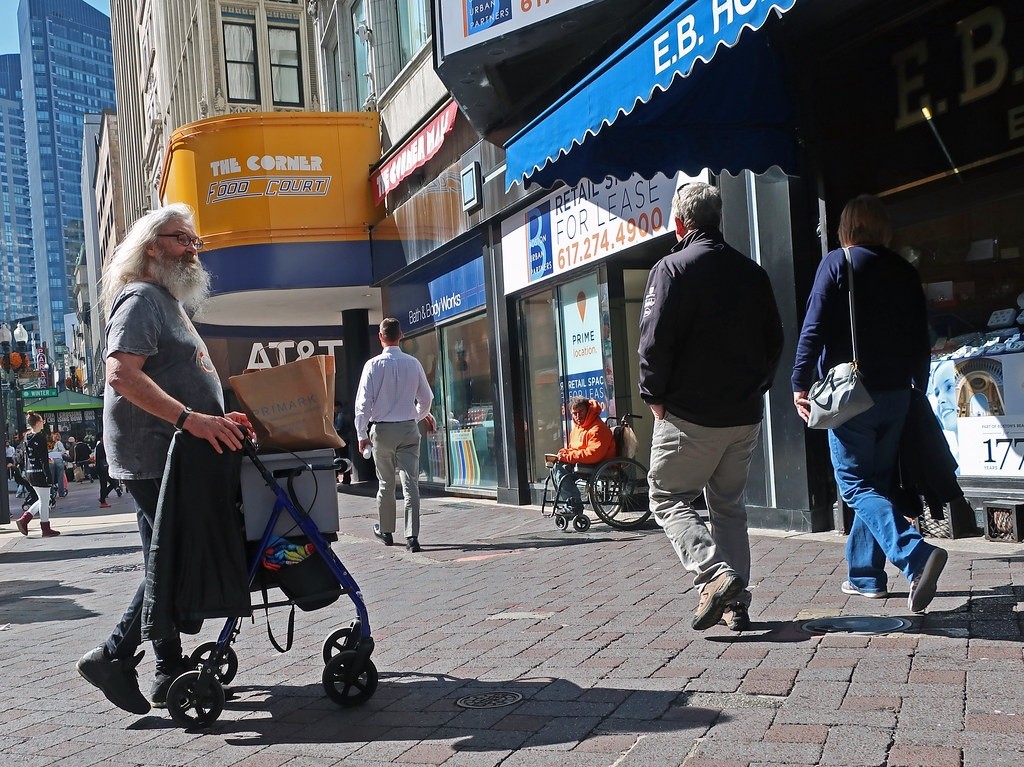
[240,448,339,542]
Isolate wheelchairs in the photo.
[541,412,652,532]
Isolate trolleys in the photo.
[164,425,380,730]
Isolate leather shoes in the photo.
[373,523,394,546]
[406,537,421,553]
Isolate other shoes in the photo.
[100,501,111,508]
[556,507,584,520]
[116,489,122,497]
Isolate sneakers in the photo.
[150,655,234,709]
[842,580,887,599]
[691,571,744,631]
[907,543,948,612]
[717,605,750,631]
[75,642,151,714]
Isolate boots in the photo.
[40,521,61,537]
[16,512,33,536]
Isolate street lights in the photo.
[1,320,29,471]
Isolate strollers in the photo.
[11,466,56,511]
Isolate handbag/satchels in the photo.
[808,362,875,429]
[227,338,349,451]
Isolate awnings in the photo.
[503,0,806,195]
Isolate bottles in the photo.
[363,444,372,459]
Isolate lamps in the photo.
[921,106,965,186]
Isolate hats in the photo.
[68,437,75,443]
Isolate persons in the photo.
[334,401,345,433]
[354,318,434,552]
[788,195,949,612]
[551,396,617,516]
[16,410,61,538]
[75,204,256,716]
[5,432,129,508]
[636,182,786,631]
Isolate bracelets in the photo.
[172,405,192,432]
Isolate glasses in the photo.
[38,420,45,424]
[153,232,203,250]
